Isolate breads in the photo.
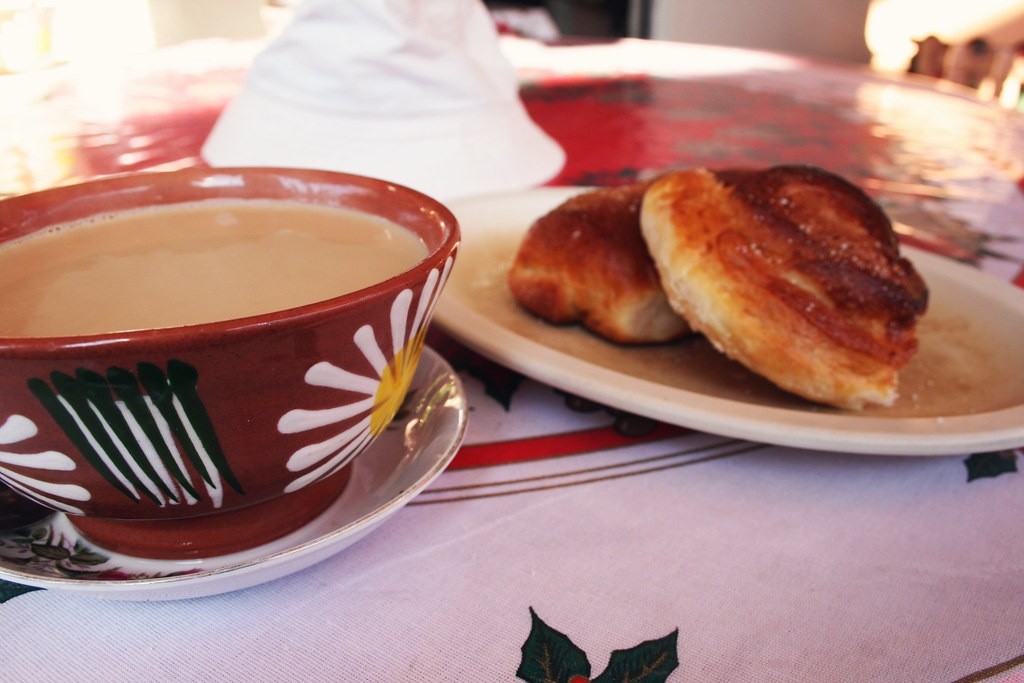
[638,160,932,412]
[510,179,702,346]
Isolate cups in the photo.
[0,166,460,560]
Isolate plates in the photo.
[0,341,470,603]
[426,187,1024,456]
